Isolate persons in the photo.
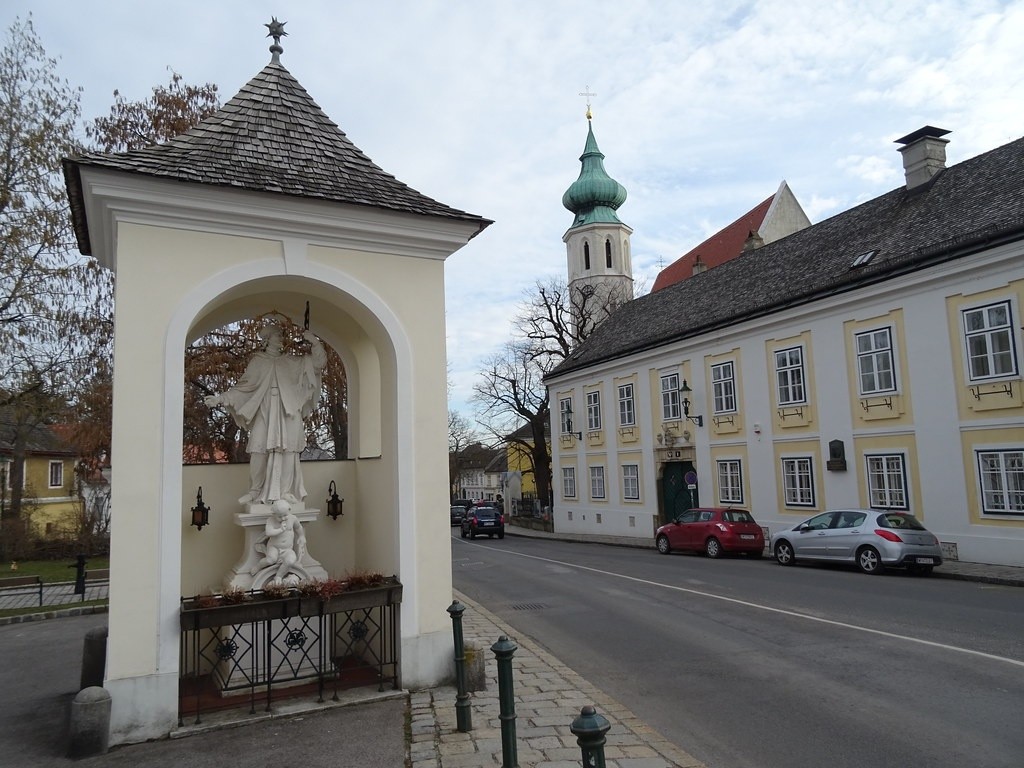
[204,324,328,506]
[252,499,307,588]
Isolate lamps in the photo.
[679,379,693,418]
[189,485,212,532]
[563,402,574,433]
[325,480,346,521]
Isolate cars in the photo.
[654,507,765,559]
[450,505,467,527]
[770,507,944,576]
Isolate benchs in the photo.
[82,569,109,602]
[0,575,44,606]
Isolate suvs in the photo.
[461,506,506,541]
